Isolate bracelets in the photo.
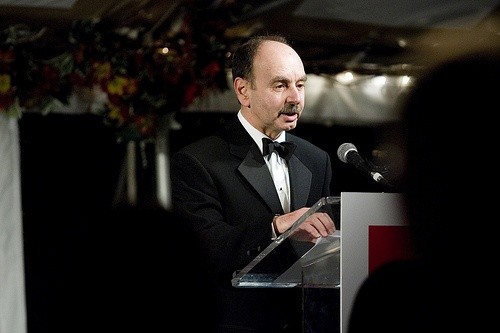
[273,214,282,237]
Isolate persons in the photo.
[171,35,332,333]
[347,49,500,333]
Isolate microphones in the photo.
[337,142,389,185]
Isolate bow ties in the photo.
[262,138,297,162]
